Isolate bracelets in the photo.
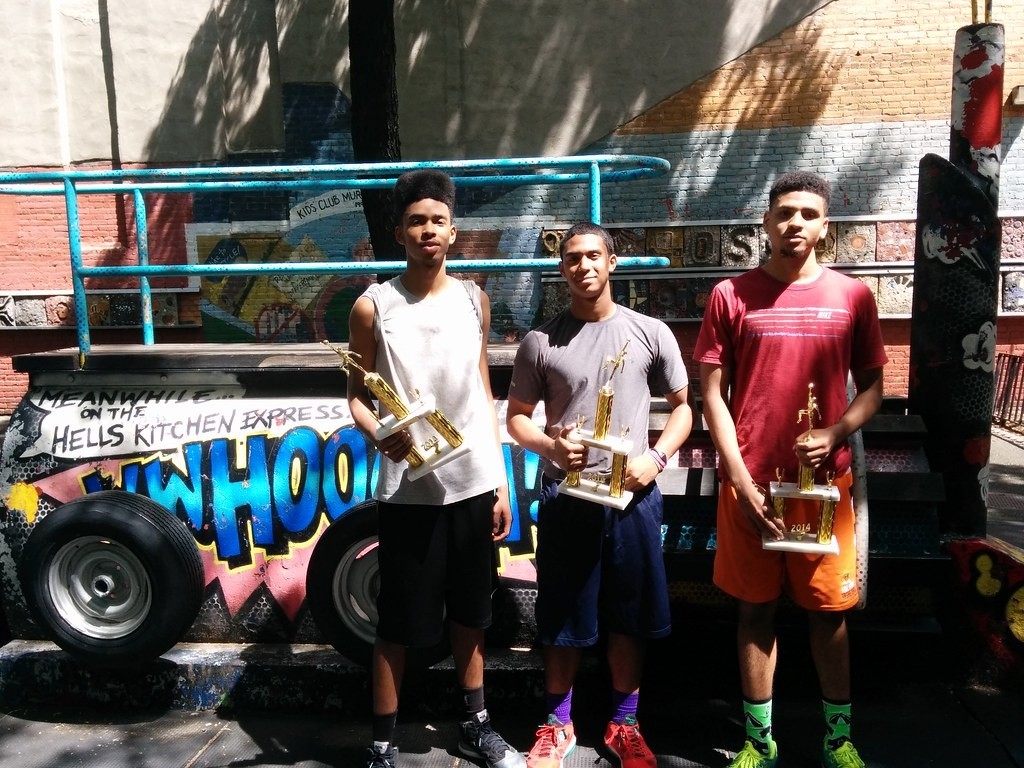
[647,448,667,473]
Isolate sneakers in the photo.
[458,709,526,768]
[822,735,866,768]
[603,719,658,768]
[726,740,778,768]
[365,740,400,768]
[526,721,577,768]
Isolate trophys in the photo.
[320,338,474,483]
[557,338,634,510]
[762,382,841,555]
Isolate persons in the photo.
[347,169,526,768]
[691,174,888,768]
[507,223,698,768]
